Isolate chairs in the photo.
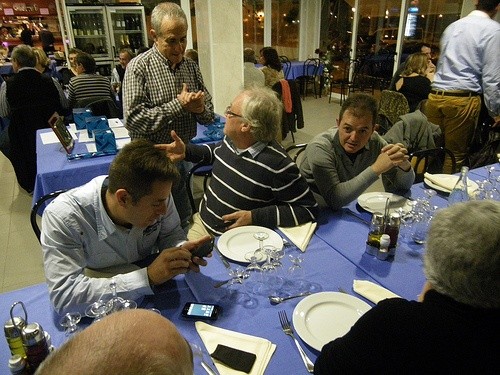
[378,91,409,135]
[329,58,375,106]
[409,147,456,184]
[297,58,319,100]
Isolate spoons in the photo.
[214,273,249,288]
[267,291,315,306]
[344,208,372,227]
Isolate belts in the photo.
[431,91,479,97]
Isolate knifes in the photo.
[211,244,230,269]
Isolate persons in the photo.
[30,46,63,82]
[423,0,500,174]
[36,308,194,375]
[298,92,418,211]
[154,88,320,246]
[392,52,432,114]
[392,42,436,80]
[111,48,136,97]
[312,198,500,375]
[242,47,266,90]
[183,48,200,63]
[259,46,283,90]
[124,2,217,230]
[37,141,214,321]
[66,51,121,119]
[1,44,67,189]
[21,23,36,47]
[38,22,56,55]
[54,47,87,90]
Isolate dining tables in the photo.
[0,229,387,375]
[37,113,228,205]
[314,163,500,301]
[253,60,324,97]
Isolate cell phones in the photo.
[181,302,220,321]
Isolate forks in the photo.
[269,226,295,249]
[278,310,316,373]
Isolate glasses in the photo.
[224,106,242,118]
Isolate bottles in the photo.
[447,165,469,206]
[71,12,143,75]
[365,212,401,261]
[4,317,55,375]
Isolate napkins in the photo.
[424,172,472,190]
[278,222,317,252]
[195,321,276,375]
[353,280,400,305]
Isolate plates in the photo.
[292,291,374,353]
[357,191,415,219]
[217,224,283,264]
[424,174,479,194]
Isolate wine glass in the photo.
[59,297,160,342]
[394,187,442,247]
[222,230,305,300]
[475,165,500,201]
